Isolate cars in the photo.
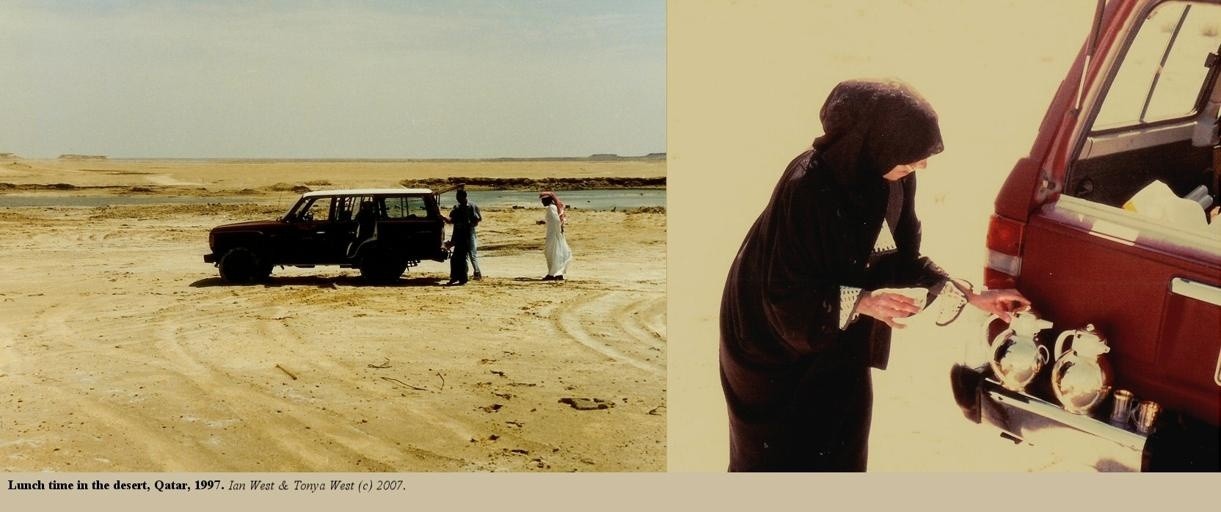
[950,0,1221,473]
[204,179,465,288]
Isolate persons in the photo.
[535,190,573,282]
[719,78,1032,471]
[441,190,482,285]
